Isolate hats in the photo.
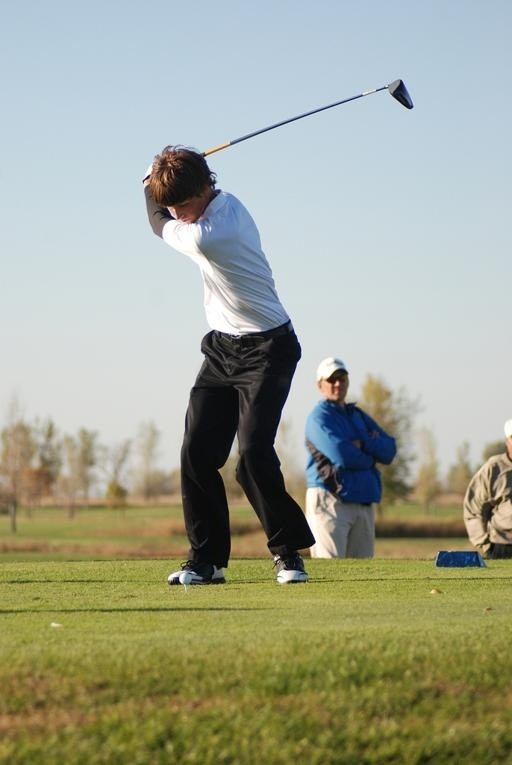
[316,357,349,383]
[503,419,512,438]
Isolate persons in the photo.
[463,420,512,559]
[306,357,396,558]
[142,144,316,585]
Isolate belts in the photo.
[214,322,294,344]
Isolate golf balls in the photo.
[179,572,192,585]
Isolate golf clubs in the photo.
[199,79,413,156]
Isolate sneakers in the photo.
[167,560,225,584]
[272,550,308,584]
